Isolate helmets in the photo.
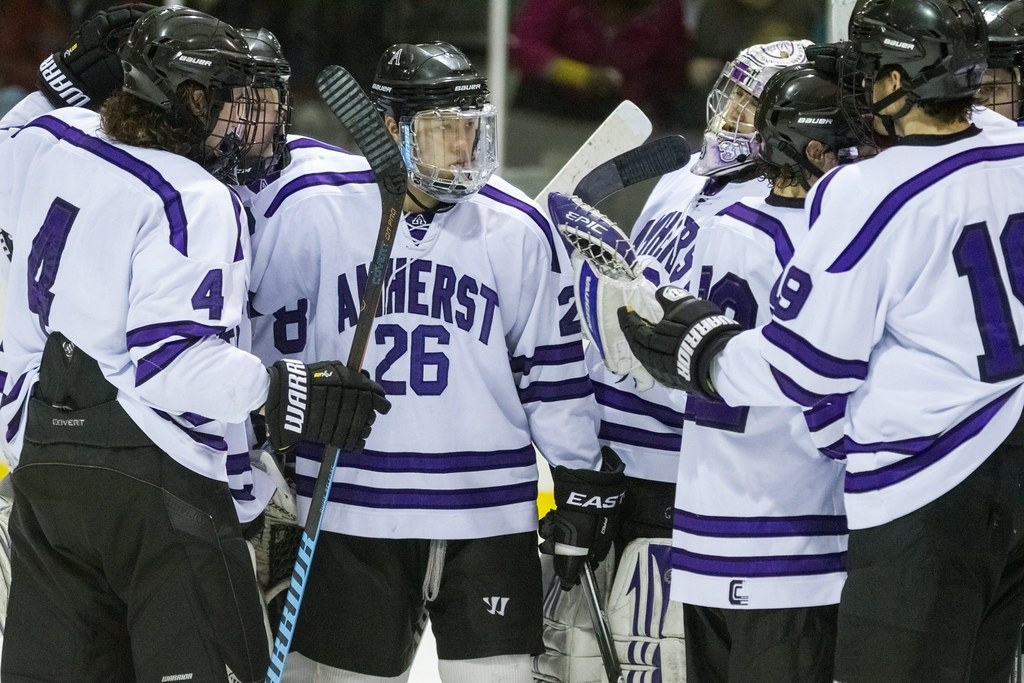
[119,4,261,181]
[974,0,1024,123]
[690,40,815,176]
[237,27,291,176]
[835,0,988,153]
[755,64,867,154]
[371,41,498,204]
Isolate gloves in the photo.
[617,285,744,402]
[538,445,626,591]
[806,42,865,88]
[35,3,157,112]
[265,359,392,455]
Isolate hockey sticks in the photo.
[579,560,629,682]
[573,131,694,208]
[263,62,414,682]
[536,99,656,222]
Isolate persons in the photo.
[2,0,631,682]
[583,0,1024,681]
